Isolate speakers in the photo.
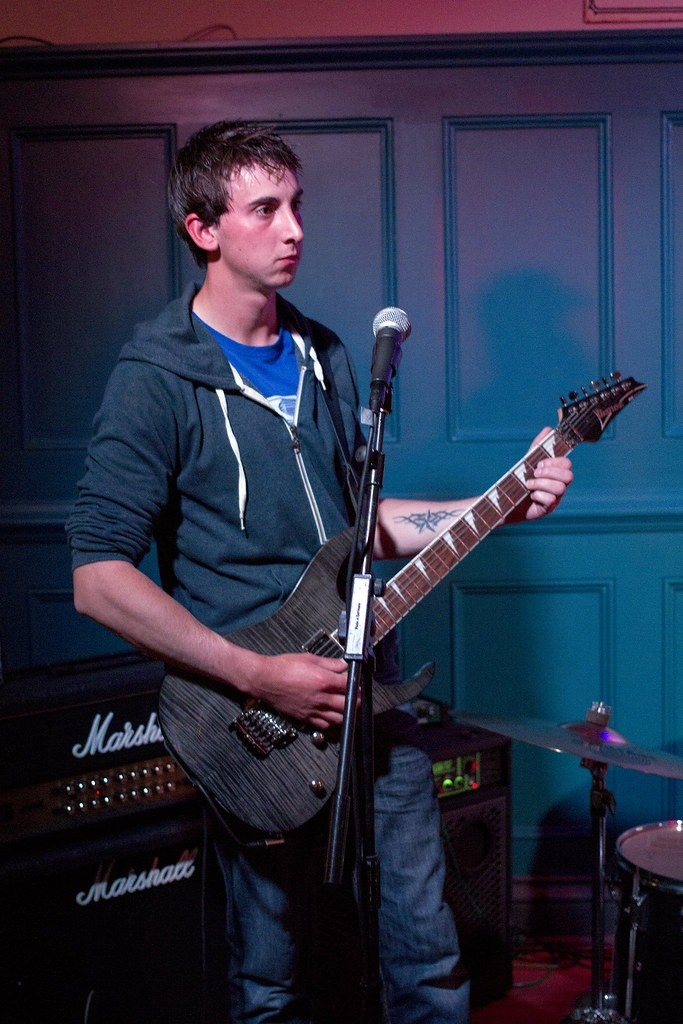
[284,695,513,1024]
[0,818,233,1024]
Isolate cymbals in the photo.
[447,708,683,781]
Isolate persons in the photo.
[64,118,574,1023]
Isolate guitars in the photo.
[155,370,649,836]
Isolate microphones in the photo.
[368,307,411,414]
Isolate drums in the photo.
[606,817,683,1024]
